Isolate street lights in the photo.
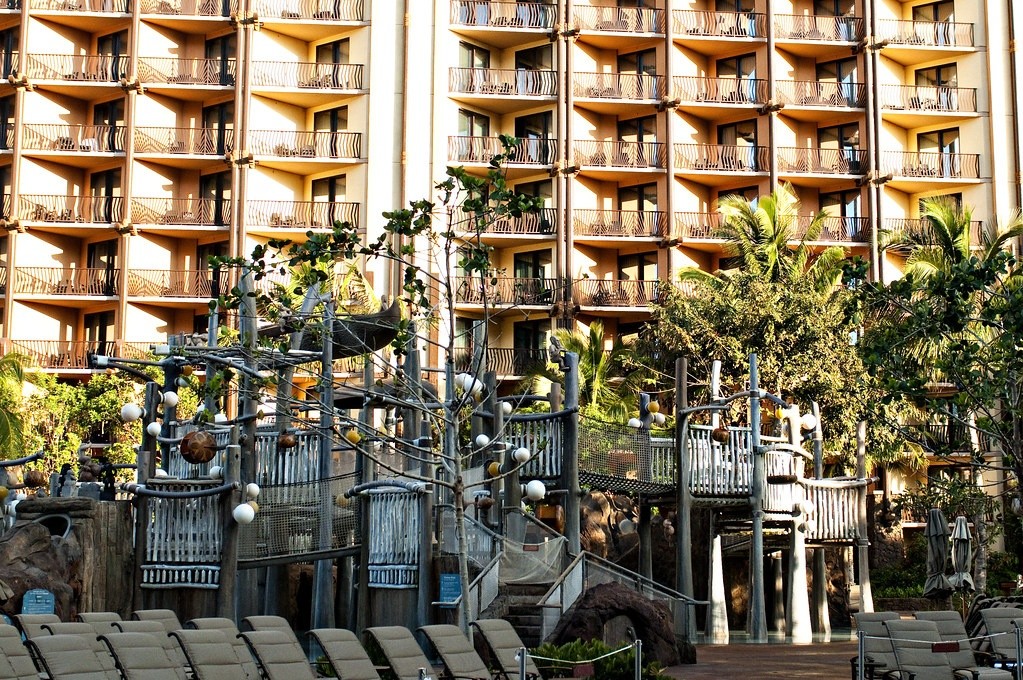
[453,317,545,541]
[617,393,666,594]
[120,334,427,624]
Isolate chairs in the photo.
[850,594,1023,680]
[0,609,544,680]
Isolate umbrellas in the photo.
[949,515,976,623]
[923,509,956,609]
